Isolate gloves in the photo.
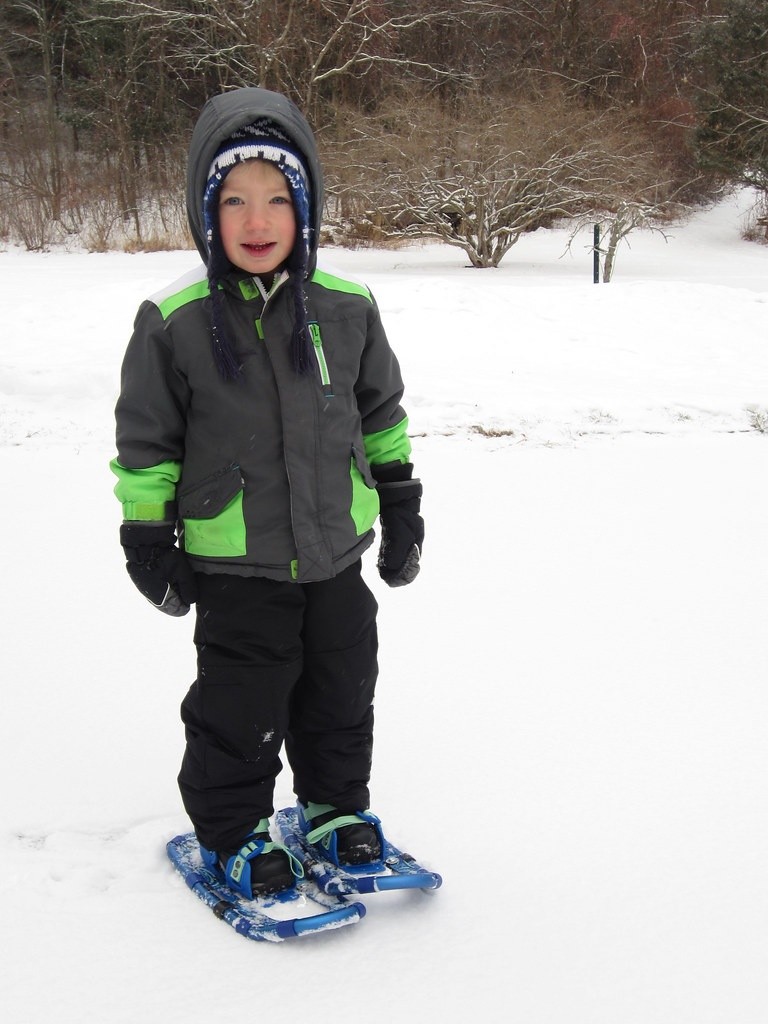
[120,521,199,617]
[375,479,424,589]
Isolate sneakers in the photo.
[298,797,383,865]
[201,832,297,893]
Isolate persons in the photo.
[110,89,425,899]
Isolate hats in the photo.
[202,117,311,381]
